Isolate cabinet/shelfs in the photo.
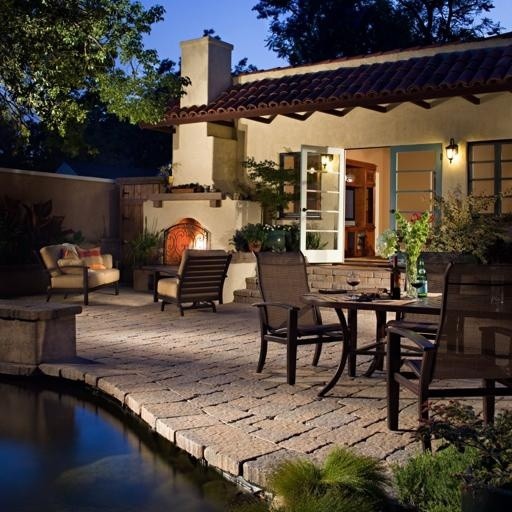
[346,158,377,257]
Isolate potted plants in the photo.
[231,154,317,253]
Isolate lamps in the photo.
[320,153,334,168]
[445,138,459,165]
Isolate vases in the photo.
[403,258,418,298]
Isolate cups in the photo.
[204,185,210,193]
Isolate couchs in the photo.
[33,244,121,305]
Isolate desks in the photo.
[299,287,512,397]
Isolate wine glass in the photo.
[345,274,360,299]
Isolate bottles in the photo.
[390,255,401,300]
[416,257,428,297]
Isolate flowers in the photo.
[377,209,430,294]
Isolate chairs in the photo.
[386,251,467,354]
[251,249,358,385]
[153,248,233,316]
[383,259,512,455]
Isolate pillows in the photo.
[60,241,80,260]
[101,254,114,268]
[76,246,102,264]
[56,258,87,273]
[87,263,106,270]
